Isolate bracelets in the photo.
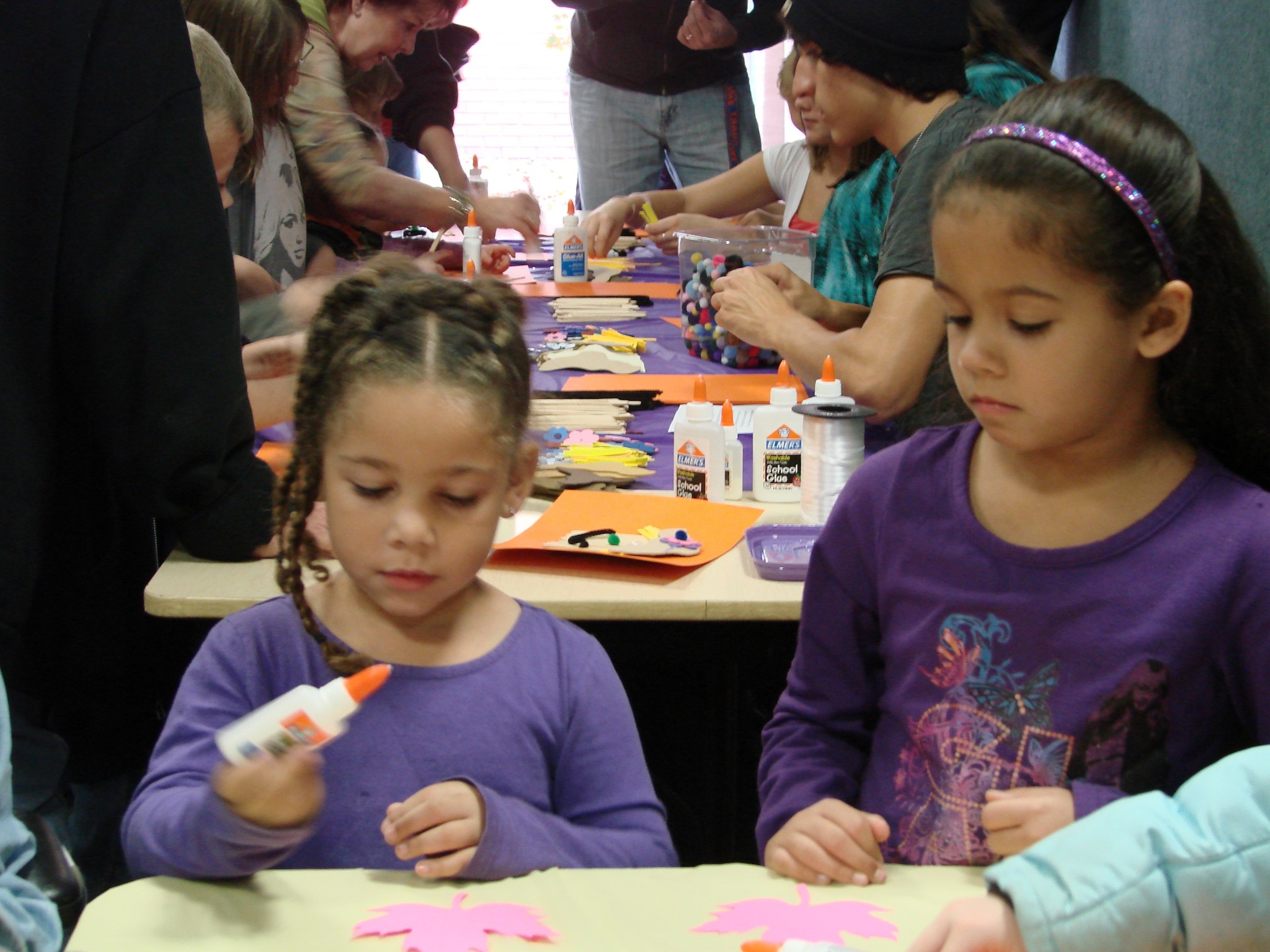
[442,186,472,227]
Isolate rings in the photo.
[685,34,693,41]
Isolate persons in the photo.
[122,254,680,884]
[255,164,306,286]
[183,18,304,434]
[380,1,476,195]
[281,0,540,245]
[554,0,787,211]
[582,48,856,265]
[753,74,1270,881]
[180,0,336,327]
[0,0,332,895]
[714,2,999,436]
[910,744,1270,951]
[812,1,1055,307]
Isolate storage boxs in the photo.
[669,225,819,372]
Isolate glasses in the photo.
[284,39,313,76]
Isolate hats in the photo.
[784,0,970,100]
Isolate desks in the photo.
[58,857,1006,952]
[139,220,875,634]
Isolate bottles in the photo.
[673,373,727,503]
[803,354,856,408]
[460,206,483,279]
[468,154,488,199]
[553,197,590,283]
[716,399,743,501]
[462,259,477,283]
[214,662,393,769]
[751,359,803,504]
